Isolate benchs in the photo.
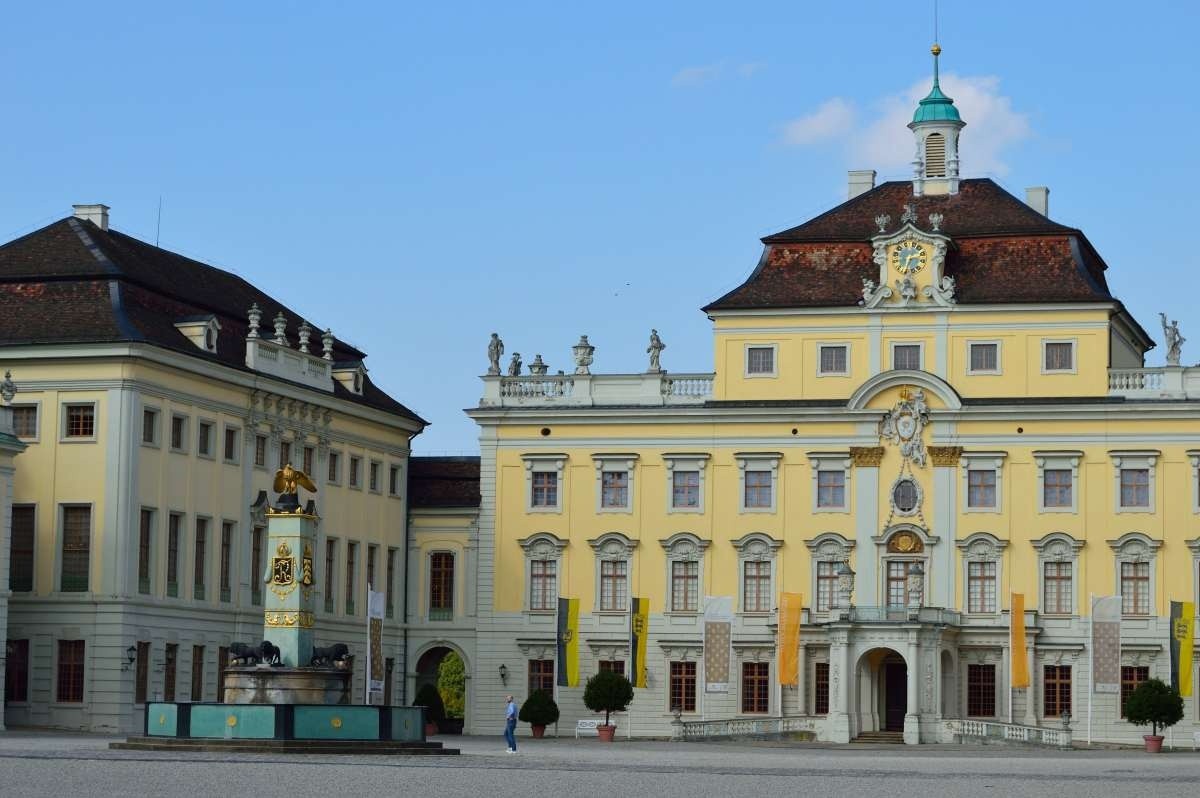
[575,720,615,740]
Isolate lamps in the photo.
[121,645,137,665]
[498,664,507,678]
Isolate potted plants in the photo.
[1124,677,1186,752]
[330,643,348,670]
[582,669,635,741]
[518,689,560,739]
[412,683,445,737]
[437,653,464,736]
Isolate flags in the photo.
[1092,597,1122,695]
[778,592,802,685]
[556,596,581,688]
[1011,593,1030,689]
[631,598,649,688]
[703,596,732,694]
[1170,601,1196,698]
[368,591,385,693]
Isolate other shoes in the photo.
[505,748,512,752]
[508,750,517,753]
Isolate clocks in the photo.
[891,241,926,274]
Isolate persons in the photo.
[1158,313,1186,366]
[647,329,665,368]
[882,389,929,468]
[487,333,504,369]
[508,352,522,376]
[504,696,518,753]
